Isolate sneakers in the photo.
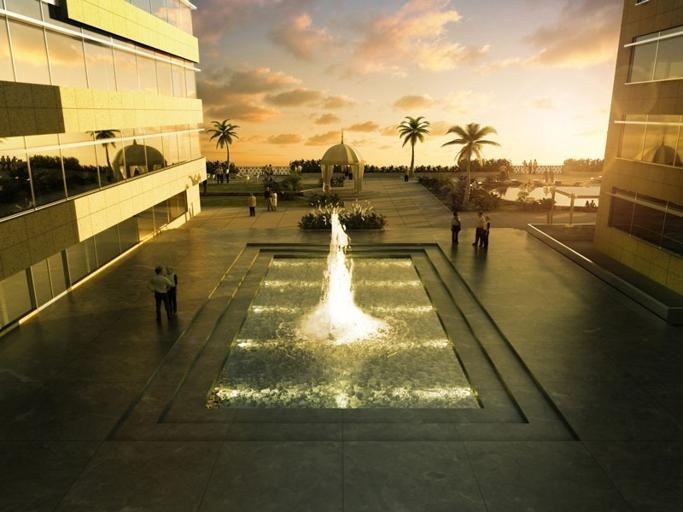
[250,213,256,217]
[452,241,458,244]
[267,209,277,212]
[217,180,229,184]
[156,312,177,320]
[472,242,488,249]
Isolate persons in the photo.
[543,168,560,184]
[481,216,490,250]
[404,166,409,182]
[249,294,251,295]
[162,265,177,316]
[589,200,596,208]
[214,166,229,185]
[450,211,461,243]
[585,200,590,208]
[521,159,538,175]
[263,186,277,212]
[247,192,256,217]
[470,211,485,247]
[145,265,172,326]
